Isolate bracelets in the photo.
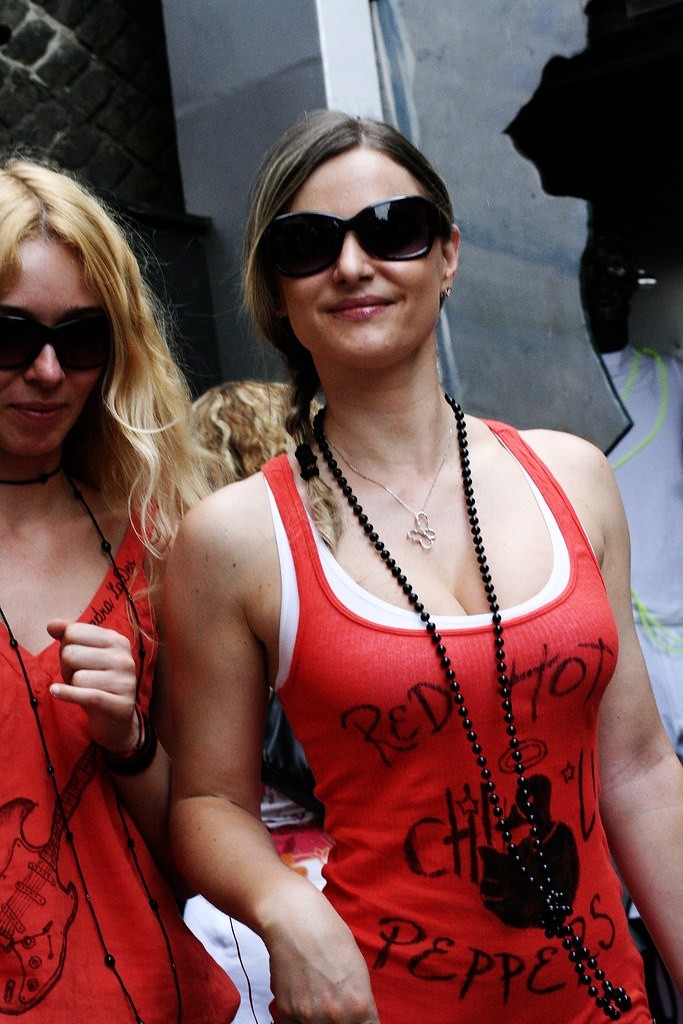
[98,706,160,774]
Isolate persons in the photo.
[0,156,244,1024]
[176,109,683,1024]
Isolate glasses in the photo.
[0,312,114,372]
[260,197,454,279]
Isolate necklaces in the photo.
[1,460,182,1024]
[323,418,455,550]
[310,390,637,1019]
